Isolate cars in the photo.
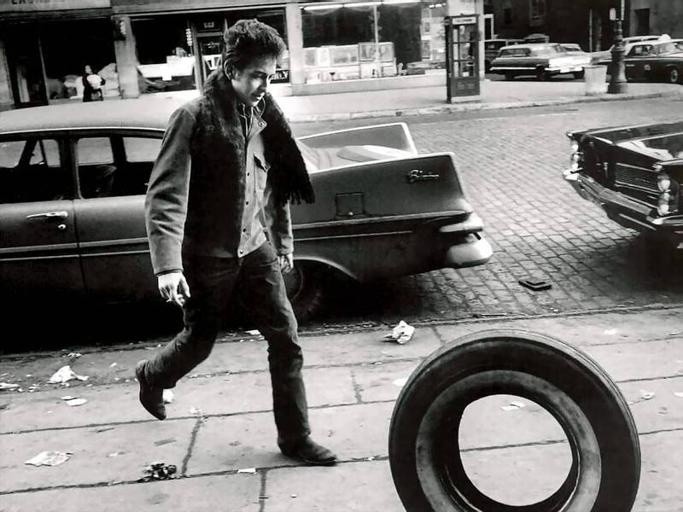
[563,123,683,258]
[491,42,591,80]
[607,39,683,84]
[590,36,661,63]
[559,44,590,65]
[0,102,493,328]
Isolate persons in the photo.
[82,64,106,102]
[134,18,337,466]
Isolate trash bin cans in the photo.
[581,65,608,96]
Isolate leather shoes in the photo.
[136,360,166,420]
[278,434,337,465]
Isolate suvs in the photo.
[462,39,528,74]
